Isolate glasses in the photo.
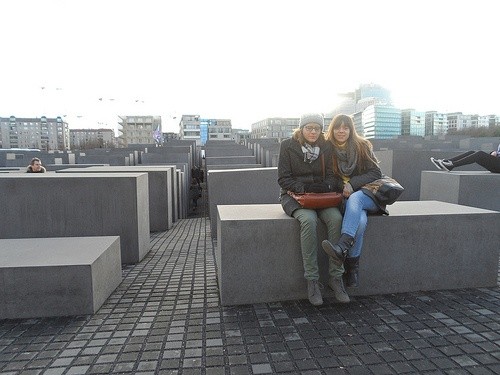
[305,126,321,133]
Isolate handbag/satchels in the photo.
[284,185,344,210]
[359,169,405,207]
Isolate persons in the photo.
[430,143,500,173]
[322,114,389,288]
[198,166,204,183]
[189,178,200,211]
[26,156,46,173]
[277,111,349,306]
[191,165,199,184]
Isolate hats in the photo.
[299,112,326,130]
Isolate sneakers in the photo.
[327,276,352,305]
[430,156,456,172]
[306,278,324,307]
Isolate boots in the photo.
[321,232,357,267]
[342,255,363,289]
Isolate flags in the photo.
[153,124,162,144]
